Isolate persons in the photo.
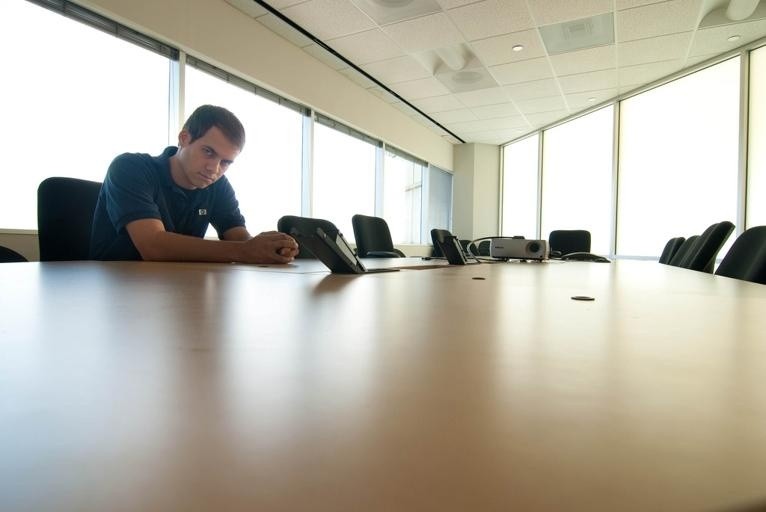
[89,105,299,264]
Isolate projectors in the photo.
[489,236,546,262]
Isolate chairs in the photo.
[547,227,592,259]
[658,218,735,274]
[35,176,104,262]
[276,215,341,257]
[352,213,405,258]
[714,223,766,284]
[427,229,490,259]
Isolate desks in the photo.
[0,258,766,512]
